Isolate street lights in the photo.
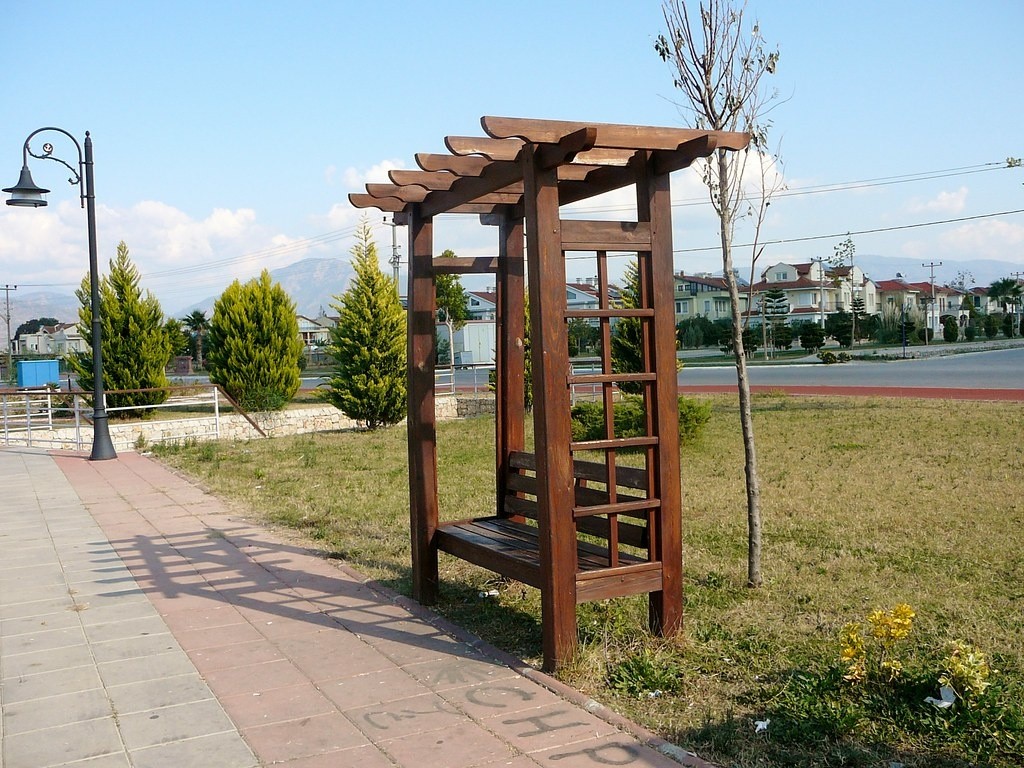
[0,125,119,461]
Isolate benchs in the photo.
[432,451,662,603]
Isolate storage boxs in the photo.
[18,360,59,387]
